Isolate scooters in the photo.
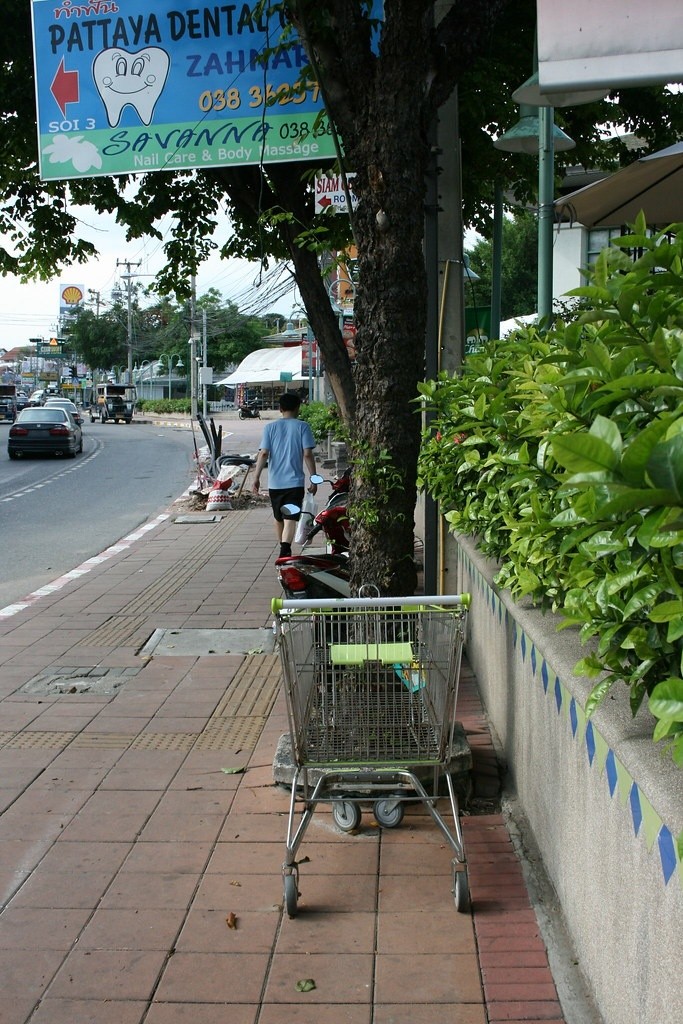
[239,398,261,420]
[274,463,359,606]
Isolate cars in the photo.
[1,382,85,457]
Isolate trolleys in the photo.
[271,590,472,922]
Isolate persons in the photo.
[251,393,317,567]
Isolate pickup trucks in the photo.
[88,395,133,425]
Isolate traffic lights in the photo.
[29,338,42,342]
[68,366,74,377]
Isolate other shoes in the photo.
[276,548,293,566]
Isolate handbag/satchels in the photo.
[295,489,318,545]
